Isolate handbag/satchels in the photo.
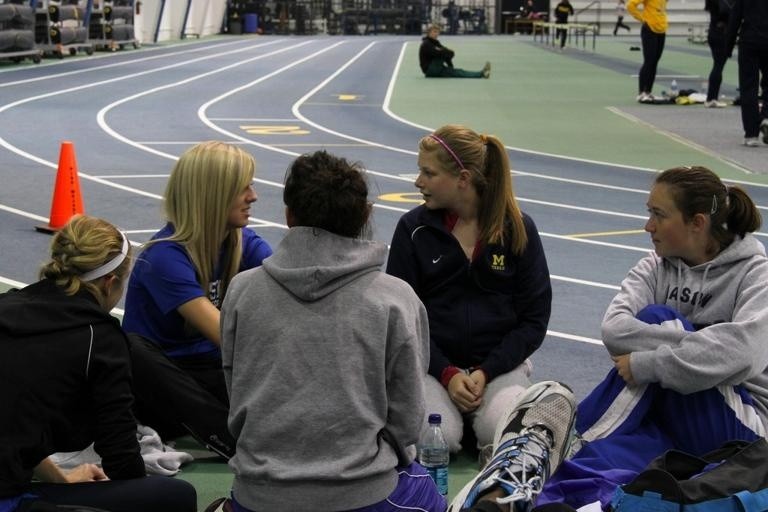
[600,437,767,512]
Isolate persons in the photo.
[705,1,767,146]
[613,1,630,35]
[626,1,668,102]
[419,25,490,79]
[515,0,574,48]
[113,138,275,459]
[406,1,488,34]
[385,122,554,465]
[0,214,199,510]
[533,160,767,510]
[217,152,451,511]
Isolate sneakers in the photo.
[636,93,651,102]
[482,63,490,77]
[759,118,767,143]
[703,99,726,107]
[742,137,759,146]
[181,419,233,461]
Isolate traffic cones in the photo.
[34,141,85,236]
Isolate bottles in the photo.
[418,414,448,502]
[671,79,678,96]
[700,80,708,95]
